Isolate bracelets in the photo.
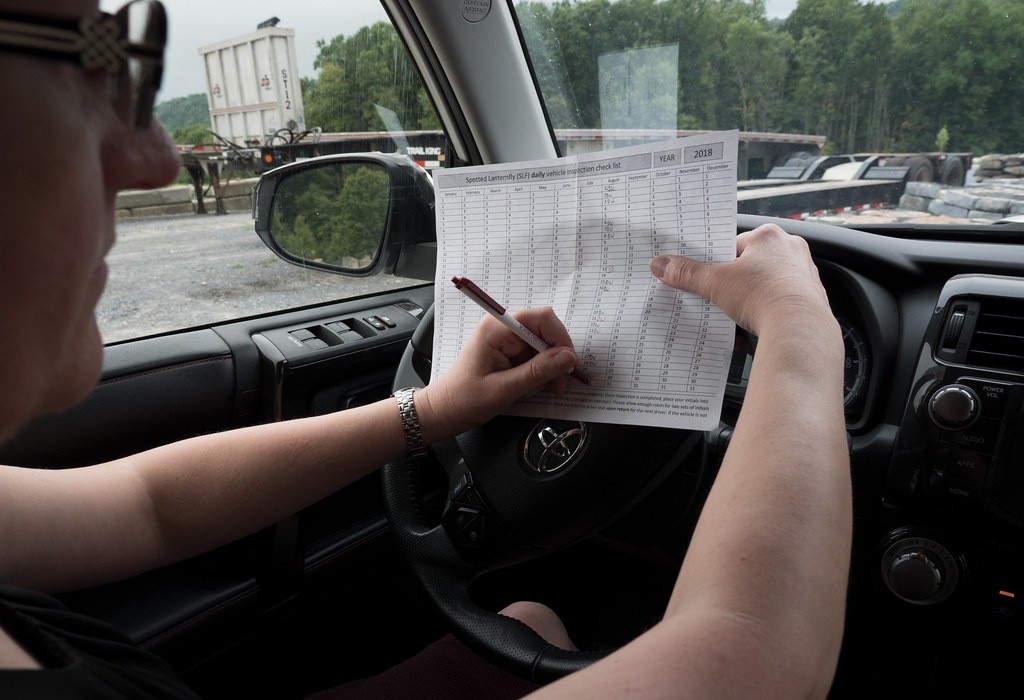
[393,387,422,440]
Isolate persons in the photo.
[0,0,852,699]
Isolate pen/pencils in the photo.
[450,275,595,387]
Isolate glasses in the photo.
[0,0,168,129]
